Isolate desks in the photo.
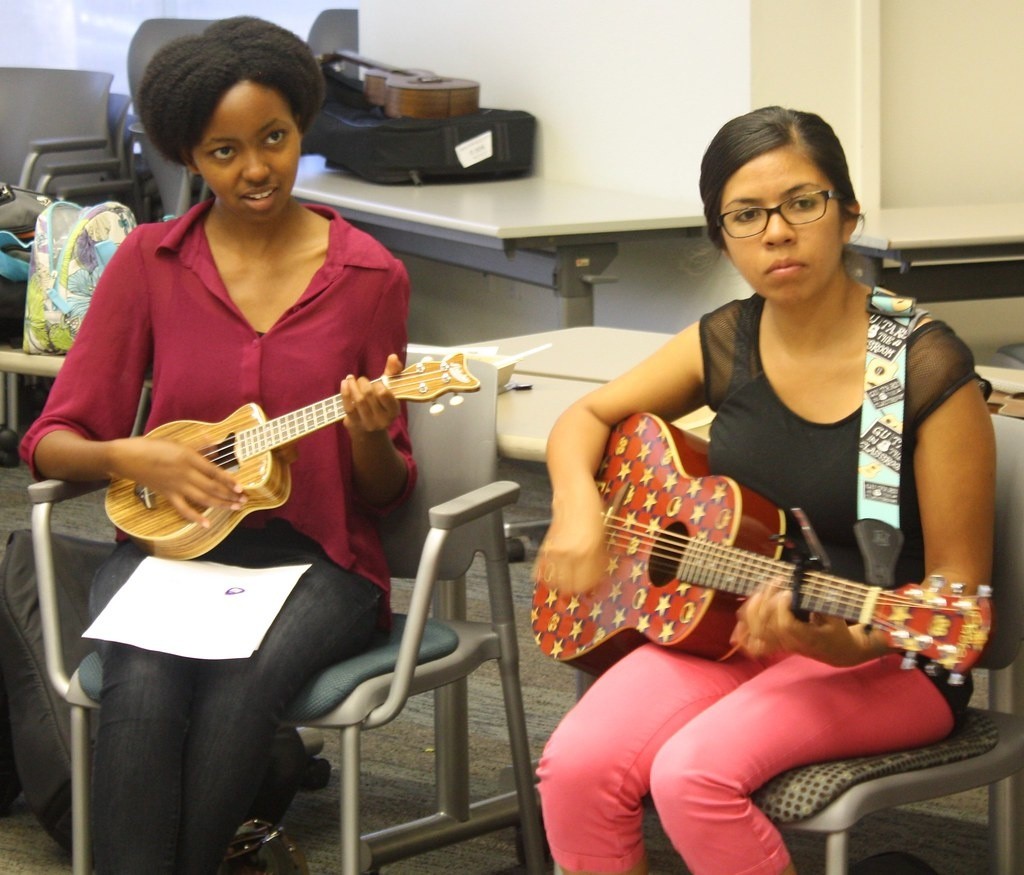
[355,326,1024,875]
[291,154,714,561]
[841,201,1024,304]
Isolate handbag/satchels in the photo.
[220,819,310,875]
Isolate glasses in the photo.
[717,189,846,238]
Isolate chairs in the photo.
[26,342,550,875]
[749,412,1024,875]
[0,7,360,468]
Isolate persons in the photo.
[526,108,999,875]
[20,17,415,875]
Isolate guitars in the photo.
[322,49,482,121]
[529,410,997,687]
[102,352,481,562]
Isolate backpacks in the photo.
[0,181,138,356]
